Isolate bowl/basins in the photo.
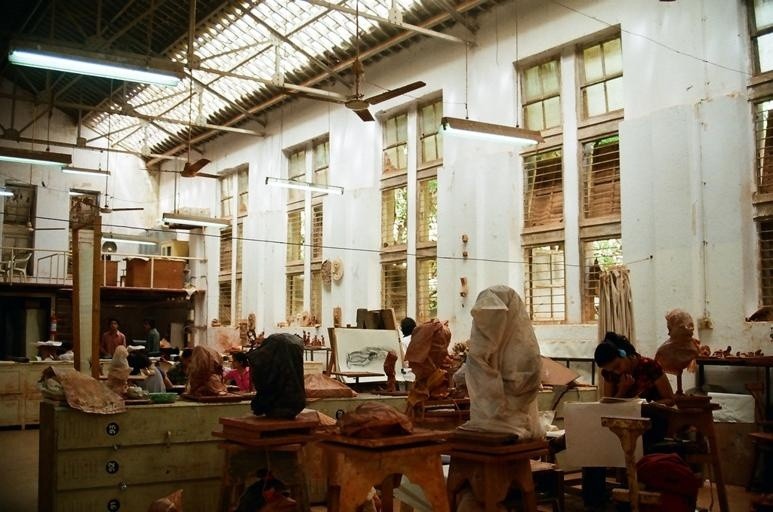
[149,392,177,402]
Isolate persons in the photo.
[223,352,255,395]
[57,341,74,361]
[141,318,160,360]
[127,354,173,394]
[593,330,674,455]
[101,319,126,360]
[167,348,193,387]
[400,318,417,359]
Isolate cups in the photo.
[539,410,555,432]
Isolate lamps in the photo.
[264,51,345,197]
[440,2,543,148]
[1,98,74,167]
[304,0,478,50]
[8,1,186,87]
[162,135,230,227]
[62,114,111,179]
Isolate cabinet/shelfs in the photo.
[0,359,414,512]
